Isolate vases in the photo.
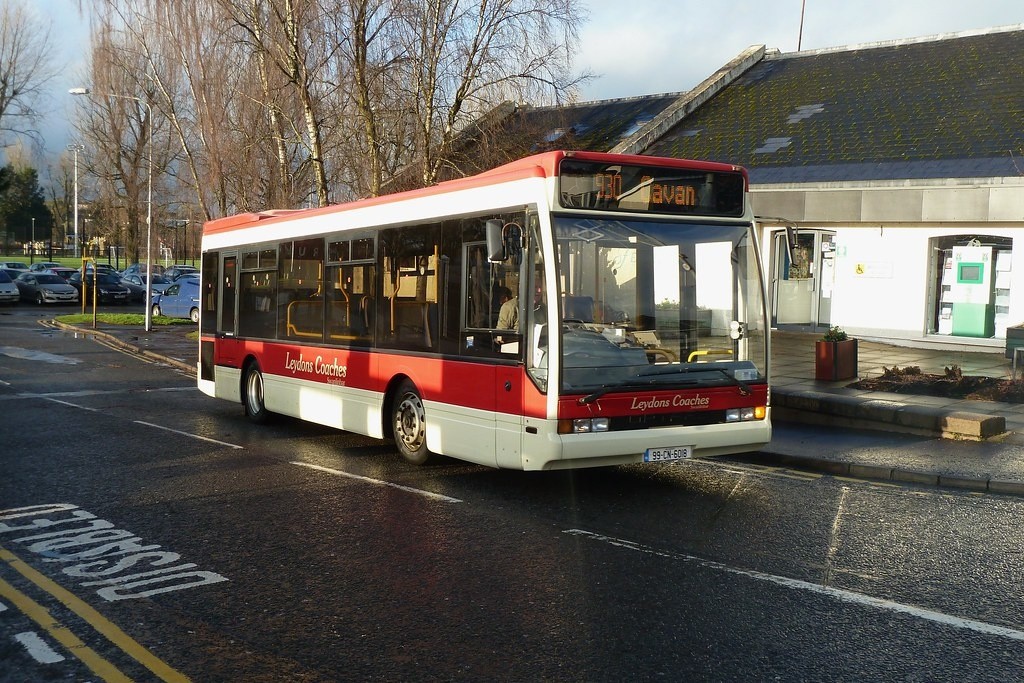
[1004,324,1024,361]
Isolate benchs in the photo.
[286,301,349,341]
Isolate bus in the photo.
[196,149,803,473]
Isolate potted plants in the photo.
[814,325,858,382]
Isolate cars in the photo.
[0,261,201,323]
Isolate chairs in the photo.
[395,297,437,348]
[563,296,594,322]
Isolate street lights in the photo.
[66,144,85,258]
[68,87,153,333]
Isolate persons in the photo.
[496,274,548,348]
